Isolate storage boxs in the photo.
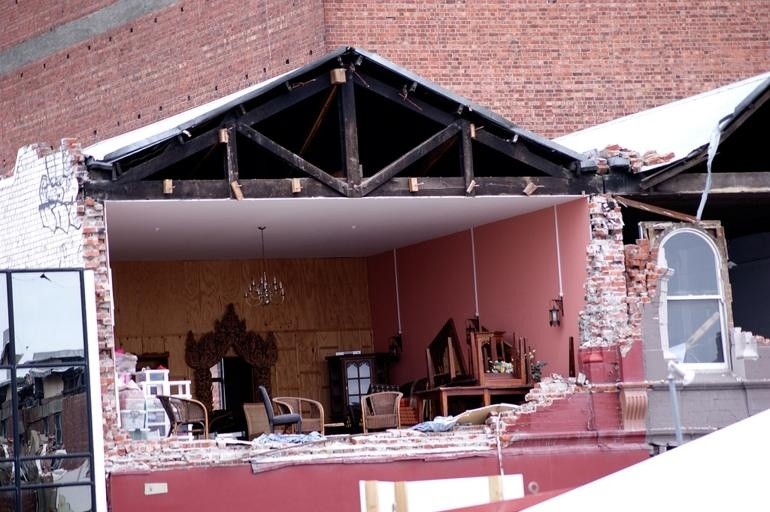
[120,389,151,443]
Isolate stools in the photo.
[425,331,575,387]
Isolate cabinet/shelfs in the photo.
[326,354,380,422]
[136,370,193,440]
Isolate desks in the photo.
[416,387,527,425]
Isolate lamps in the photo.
[244,226,286,308]
[549,299,564,327]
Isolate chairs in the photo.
[360,390,403,434]
[156,395,209,441]
[243,385,325,437]
